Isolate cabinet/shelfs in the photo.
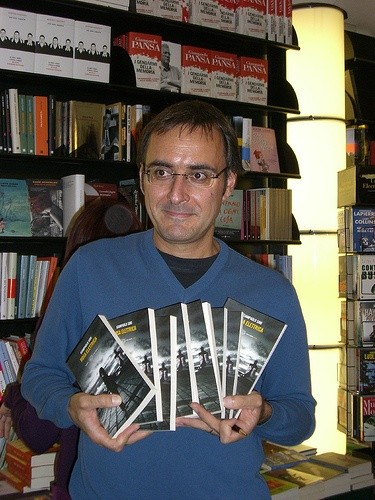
[0,0,302,332]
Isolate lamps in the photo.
[273,2,346,456]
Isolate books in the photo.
[0,333,34,405]
[335,69,375,450]
[0,253,61,320]
[0,174,150,237]
[247,253,292,283]
[215,189,292,242]
[231,116,281,173]
[0,88,150,164]
[0,0,293,108]
[0,428,60,496]
[259,440,375,500]
[65,297,288,441]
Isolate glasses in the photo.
[142,165,226,188]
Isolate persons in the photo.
[20,100,317,500]
